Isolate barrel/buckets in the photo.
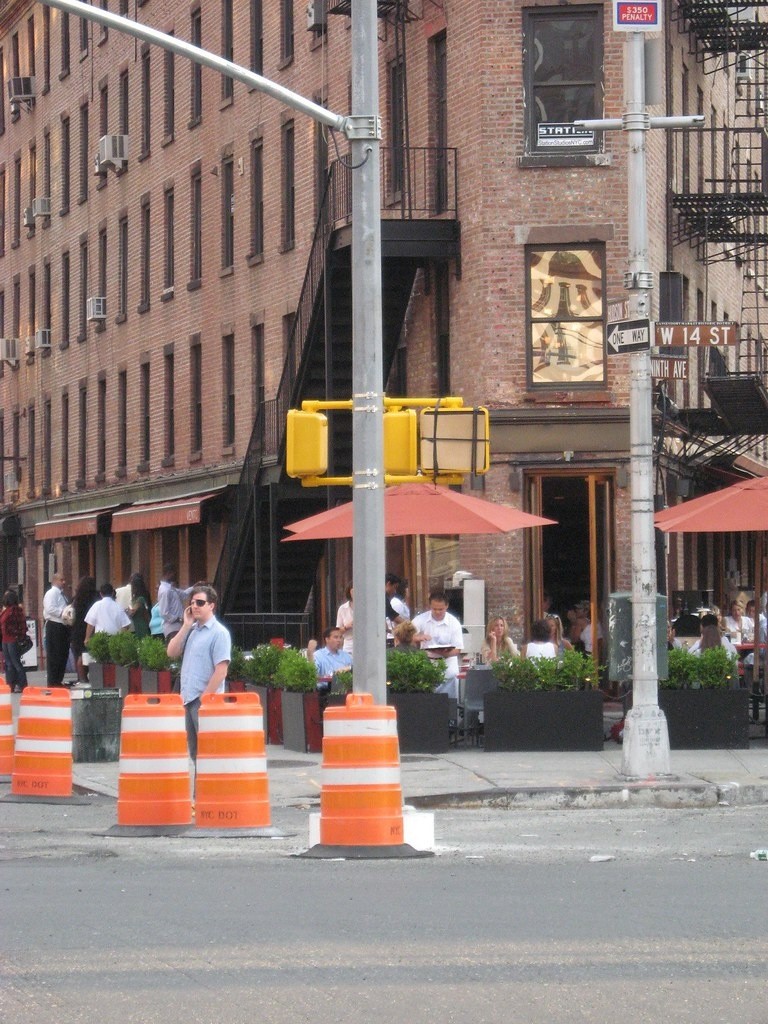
[71,697,122,762]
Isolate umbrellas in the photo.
[279,482,559,617]
[654,473,768,718]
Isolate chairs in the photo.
[464,663,493,752]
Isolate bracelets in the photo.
[344,625,348,630]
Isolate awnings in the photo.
[35,490,224,540]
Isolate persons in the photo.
[335,580,354,653]
[545,614,572,657]
[149,585,165,641]
[388,620,428,657]
[41,573,70,690]
[0,589,28,693]
[306,628,353,691]
[71,572,153,682]
[167,586,232,816]
[385,574,411,639]
[540,588,604,656]
[481,616,520,666]
[158,564,206,694]
[411,593,465,699]
[521,620,559,661]
[668,589,768,690]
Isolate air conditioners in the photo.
[737,52,754,81]
[31,196,51,217]
[86,296,107,322]
[35,329,51,349]
[99,135,128,166]
[6,76,36,103]
[25,335,35,356]
[0,337,19,361]
[3,471,19,492]
[23,207,35,227]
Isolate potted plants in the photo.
[483,644,607,752]
[626,639,751,750]
[225,643,245,691]
[129,632,173,695]
[317,645,452,754]
[270,646,323,753]
[230,642,284,746]
[85,630,138,694]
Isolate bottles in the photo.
[470,654,476,670]
[743,633,748,644]
[674,596,682,618]
[683,595,690,615]
[682,640,689,653]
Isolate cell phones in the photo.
[189,608,192,614]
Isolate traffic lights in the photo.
[285,409,330,479]
[379,408,419,477]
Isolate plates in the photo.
[428,645,452,648]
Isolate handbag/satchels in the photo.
[15,634,33,657]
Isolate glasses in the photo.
[546,613,559,619]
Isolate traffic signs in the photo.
[604,317,651,357]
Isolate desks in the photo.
[733,642,765,661]
[455,673,467,704]
[318,673,332,693]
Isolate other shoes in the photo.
[14,685,23,692]
